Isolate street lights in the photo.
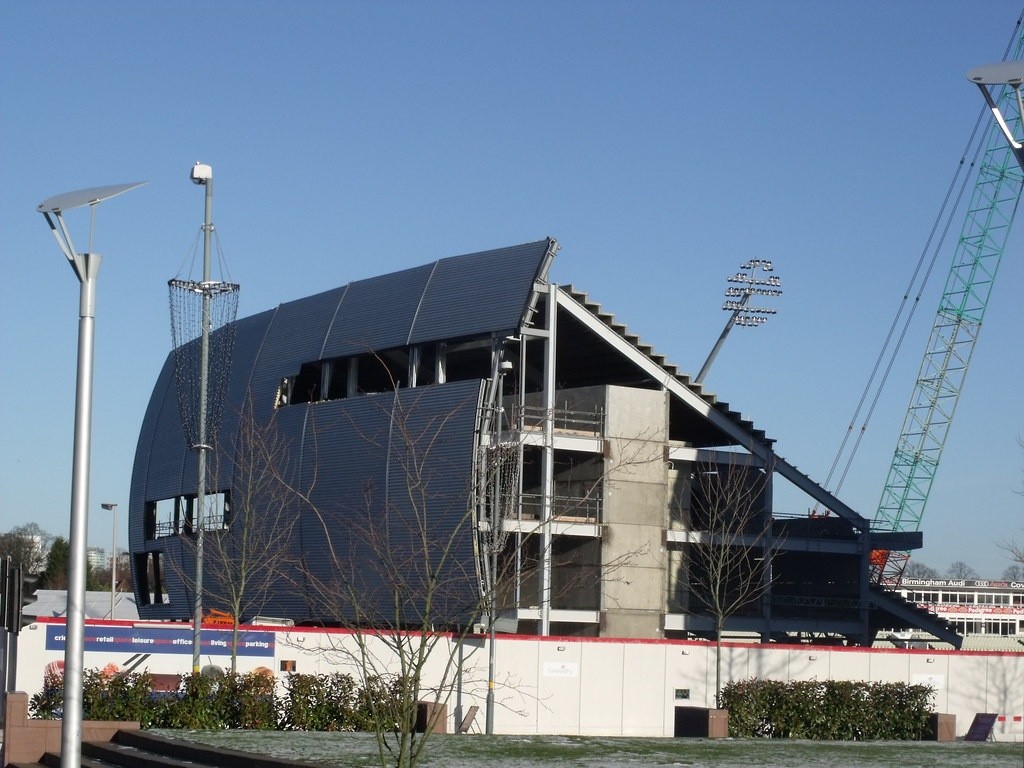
[101,503,118,620]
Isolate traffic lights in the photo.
[13,564,39,636]
